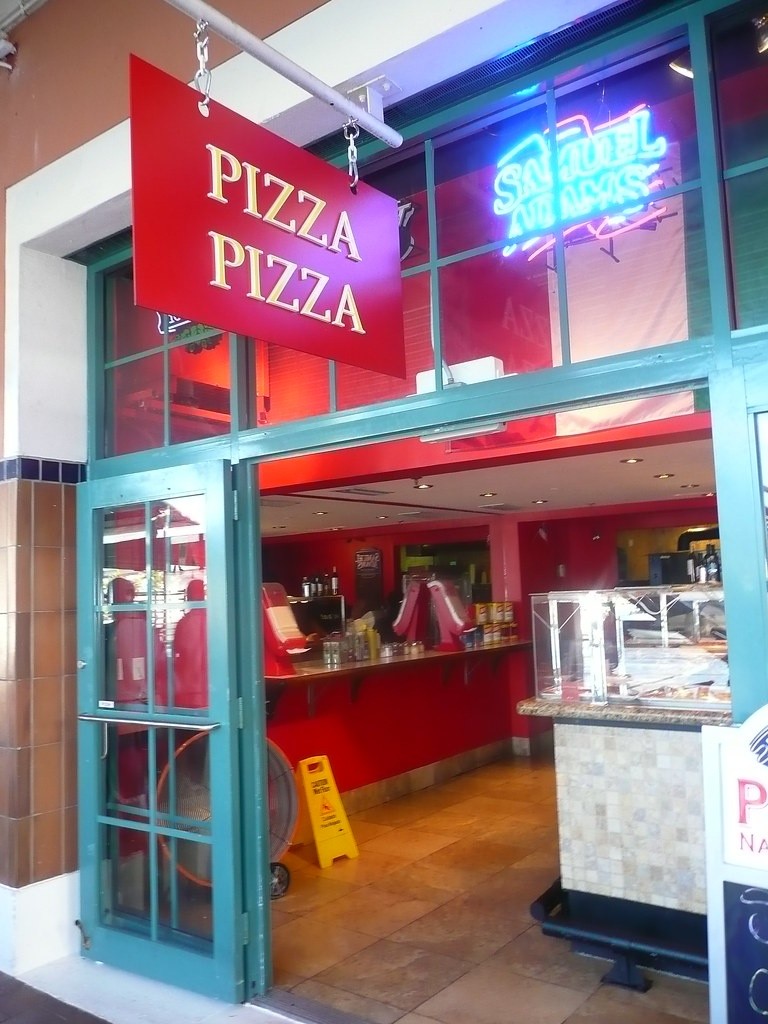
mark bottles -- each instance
[301,566,338,597]
[686,544,720,583]
[381,641,424,657]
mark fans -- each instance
[155,730,300,888]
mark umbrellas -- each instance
[103,501,205,571]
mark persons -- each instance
[174,579,208,785]
[107,578,160,804]
